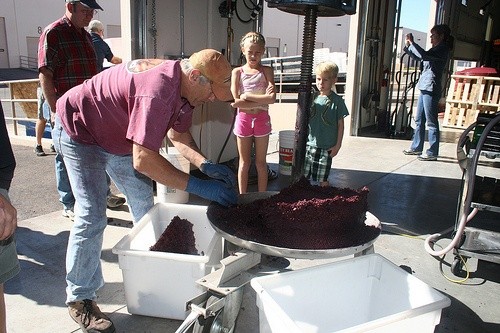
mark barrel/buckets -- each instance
[278,130,295,176]
[156,146,190,203]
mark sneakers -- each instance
[105,193,126,207]
[34,146,45,155]
[50,145,55,152]
[63,207,75,222]
[67,298,115,333]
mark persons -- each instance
[33,79,55,156]
[50,48,233,333]
[84,21,122,72]
[0,100,23,333]
[403,23,454,160]
[301,60,350,187]
[230,32,276,195]
[37,0,126,221]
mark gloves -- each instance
[186,174,239,208]
[199,161,237,187]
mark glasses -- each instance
[80,2,97,14]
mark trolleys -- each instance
[451,105,500,276]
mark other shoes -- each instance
[418,153,436,161]
[403,149,422,154]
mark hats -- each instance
[64,0,103,11]
[189,49,235,104]
